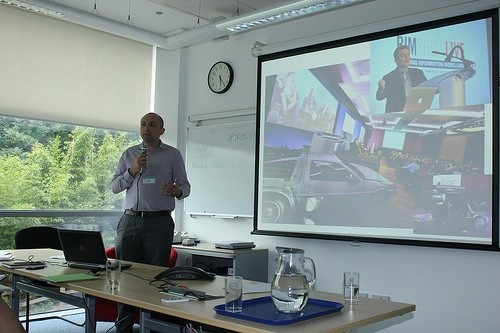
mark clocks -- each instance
[208,61,234,94]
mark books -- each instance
[213,241,255,249]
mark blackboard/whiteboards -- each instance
[185,119,255,219]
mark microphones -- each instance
[431,45,476,66]
[139,143,147,174]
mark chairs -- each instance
[15,226,63,333]
[95,246,178,322]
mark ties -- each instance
[401,70,411,96]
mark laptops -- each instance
[57,229,133,271]
[403,84,437,111]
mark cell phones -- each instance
[27,266,44,270]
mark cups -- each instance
[106,260,120,290]
[224,276,242,312]
[344,272,359,303]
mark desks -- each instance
[0,248,416,333]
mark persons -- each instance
[111,112,190,333]
[0,293,26,333]
[376,46,427,112]
[389,152,480,187]
[276,73,330,127]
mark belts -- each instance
[124,210,171,218]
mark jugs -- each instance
[271,246,316,313]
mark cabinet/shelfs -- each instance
[172,243,269,285]
